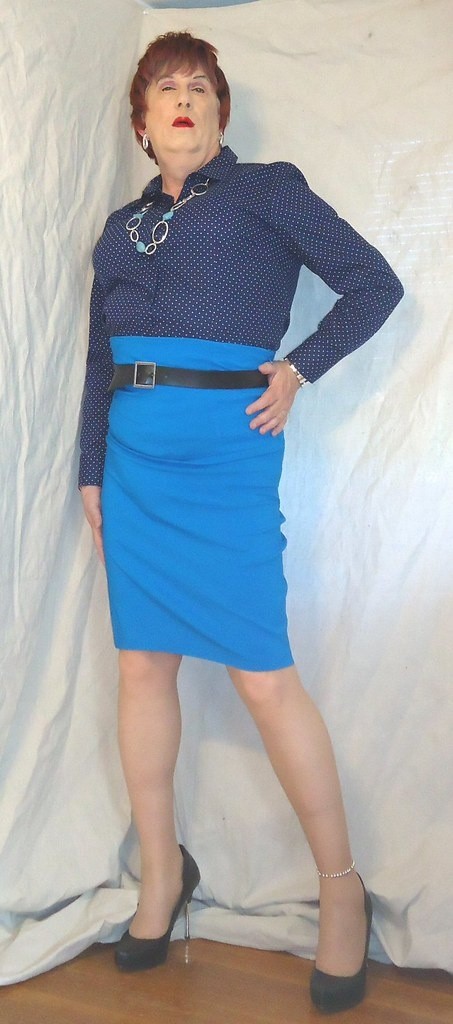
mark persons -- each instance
[76,33,405,1016]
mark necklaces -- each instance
[125,176,212,255]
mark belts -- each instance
[106,360,269,390]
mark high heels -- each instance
[308,872,373,1012]
[114,843,201,971]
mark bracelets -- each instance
[284,357,311,387]
